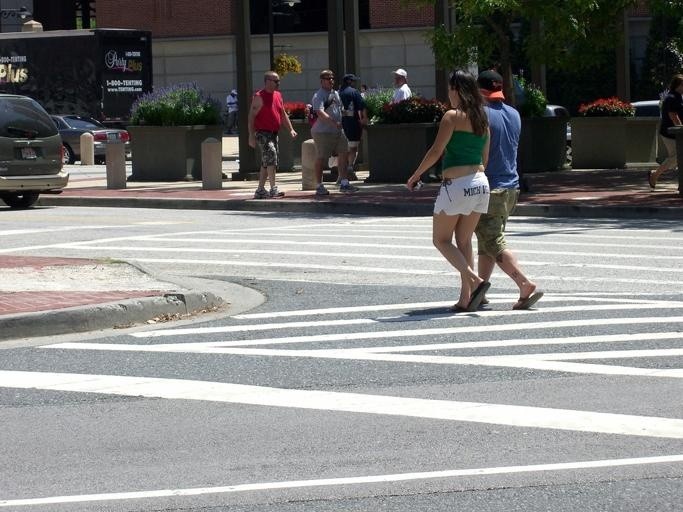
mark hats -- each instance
[474,69,506,102]
[390,68,408,80]
[342,73,361,82]
[232,89,238,96]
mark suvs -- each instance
[0,94,69,209]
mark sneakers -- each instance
[269,188,285,197]
[346,166,359,180]
[339,183,359,193]
[254,189,273,199]
[647,169,656,188]
[315,184,330,195]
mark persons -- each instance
[406,71,490,312]
[474,70,543,309]
[647,74,682,188]
[224,68,413,198]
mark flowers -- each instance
[362,83,417,120]
[284,102,306,119]
[382,97,449,123]
[580,97,636,116]
[127,81,222,126]
[513,68,550,117]
[272,53,300,78]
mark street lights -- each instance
[268,1,301,71]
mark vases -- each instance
[570,117,661,168]
[363,123,432,180]
[276,119,305,171]
[126,125,227,180]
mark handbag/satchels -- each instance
[308,97,335,127]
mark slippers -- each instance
[445,304,477,313]
[465,279,491,312]
[511,291,544,311]
[468,300,492,312]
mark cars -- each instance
[544,105,571,140]
[629,101,662,120]
[46,114,128,163]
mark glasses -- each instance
[267,78,281,84]
[320,77,335,81]
